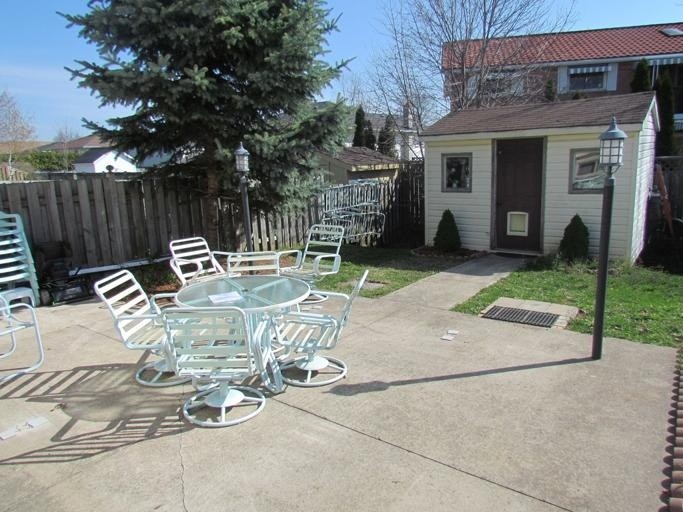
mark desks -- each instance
[173,275,312,394]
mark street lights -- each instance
[591,115,627,359]
[234,140,252,252]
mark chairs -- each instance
[94,268,198,388]
[274,223,346,306]
[268,270,368,388]
[225,251,280,276]
[158,306,266,426]
[167,237,242,287]
[1,211,46,388]
